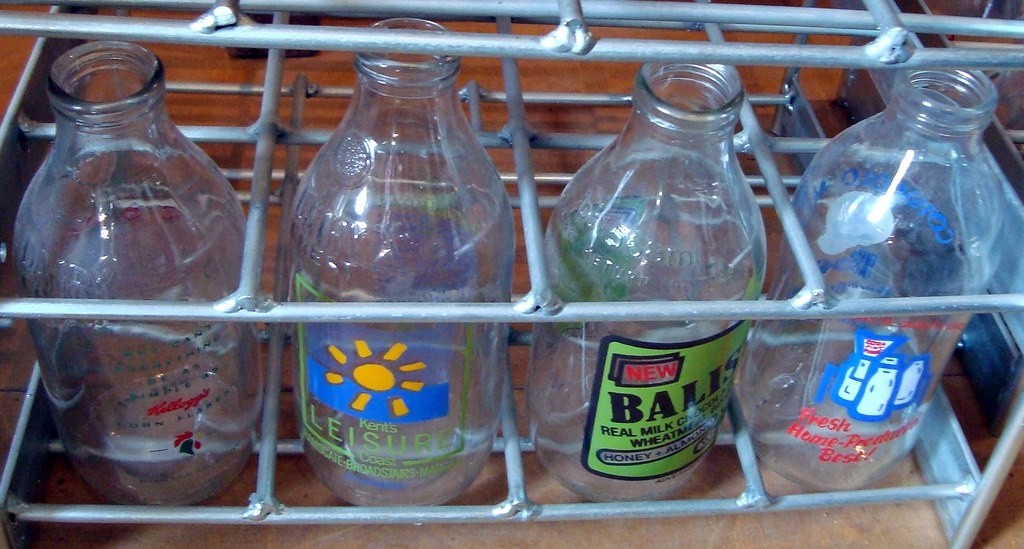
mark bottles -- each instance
[285,19,517,506]
[728,62,1009,489]
[523,63,770,500]
[11,40,263,506]
